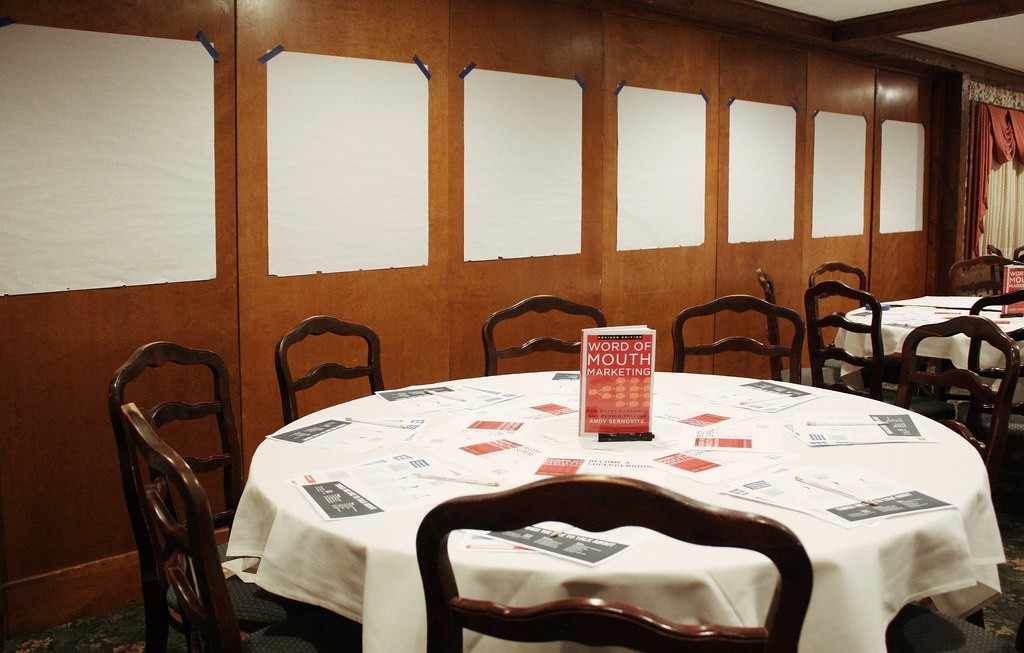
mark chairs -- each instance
[808,261,866,370]
[415,473,814,652]
[482,292,608,376]
[893,314,1021,481]
[805,278,956,420]
[885,601,1024,653]
[757,267,841,387]
[105,339,285,652]
[672,294,806,384]
[949,255,1017,295]
[956,289,1024,438]
[274,314,386,426]
[985,244,1024,300]
[122,401,363,652]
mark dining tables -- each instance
[220,368,1006,652]
[834,295,1024,403]
